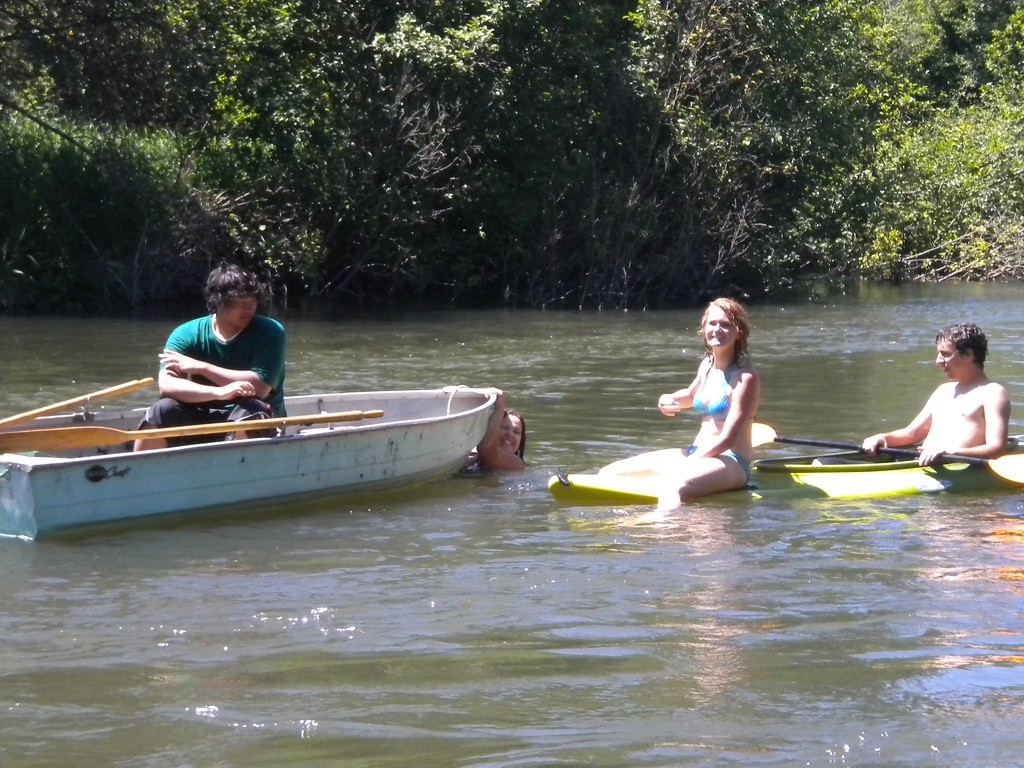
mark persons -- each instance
[130,263,288,453]
[449,386,527,482]
[658,296,761,509]
[862,321,1012,466]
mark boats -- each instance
[545,434,1024,507]
[0,383,499,543]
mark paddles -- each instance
[0,409,385,456]
[749,421,1024,484]
[0,377,155,431]
